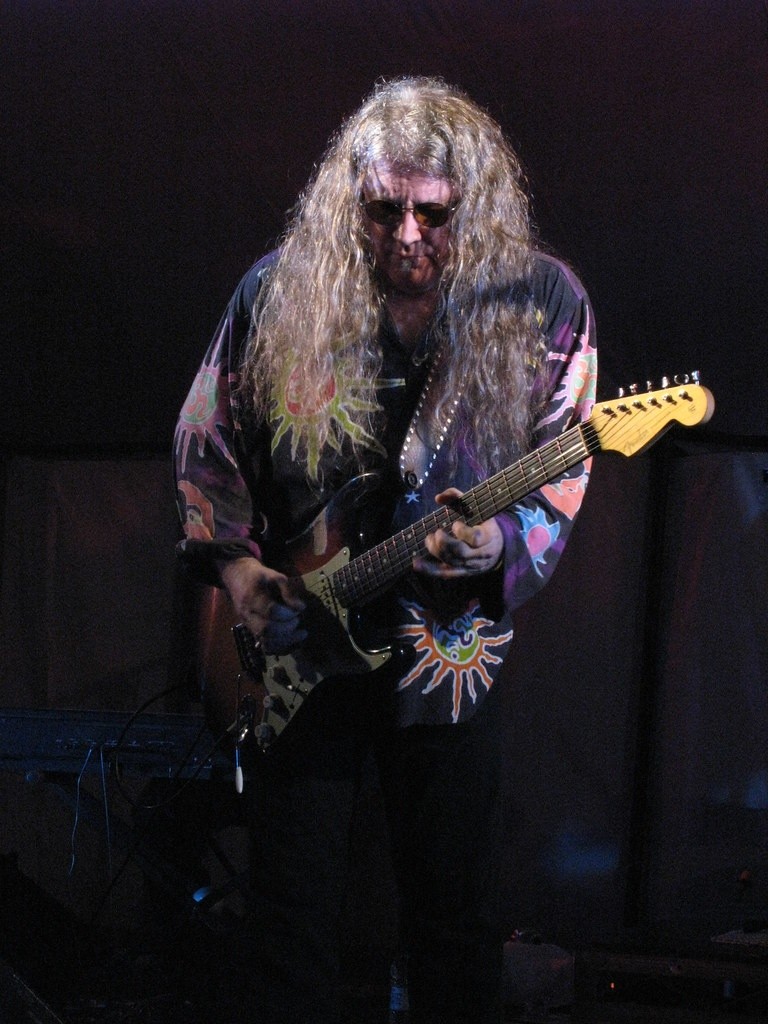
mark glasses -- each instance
[361,199,458,228]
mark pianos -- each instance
[0,706,245,932]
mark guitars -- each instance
[191,368,717,770]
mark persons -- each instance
[170,72,597,1024]
